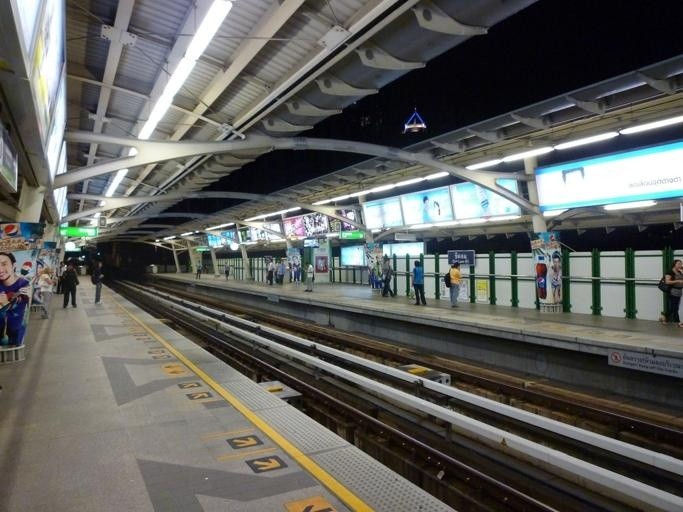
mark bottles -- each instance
[536,256,547,300]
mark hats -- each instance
[451,262,460,268]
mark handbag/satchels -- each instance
[658,278,672,292]
[445,273,452,287]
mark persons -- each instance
[195,260,201,280]
[0,252,46,344]
[40,265,56,318]
[448,261,461,308]
[224,262,230,281]
[263,257,285,285]
[91,260,104,304]
[56,261,79,308]
[305,262,314,292]
[550,252,562,303]
[381,257,396,298]
[422,196,443,221]
[411,260,427,306]
[202,264,208,274]
[288,256,301,282]
[658,260,683,328]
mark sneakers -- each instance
[660,317,683,328]
